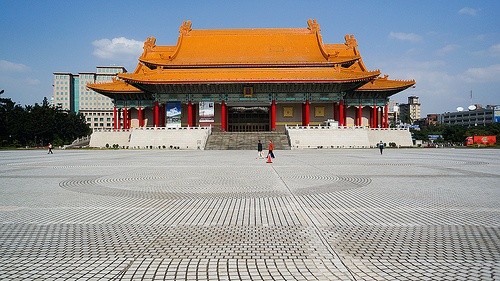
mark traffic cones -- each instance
[266,153,273,163]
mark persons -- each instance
[48,142,53,155]
[266,140,275,158]
[378,140,385,154]
[256,140,265,159]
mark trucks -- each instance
[462,135,496,147]
